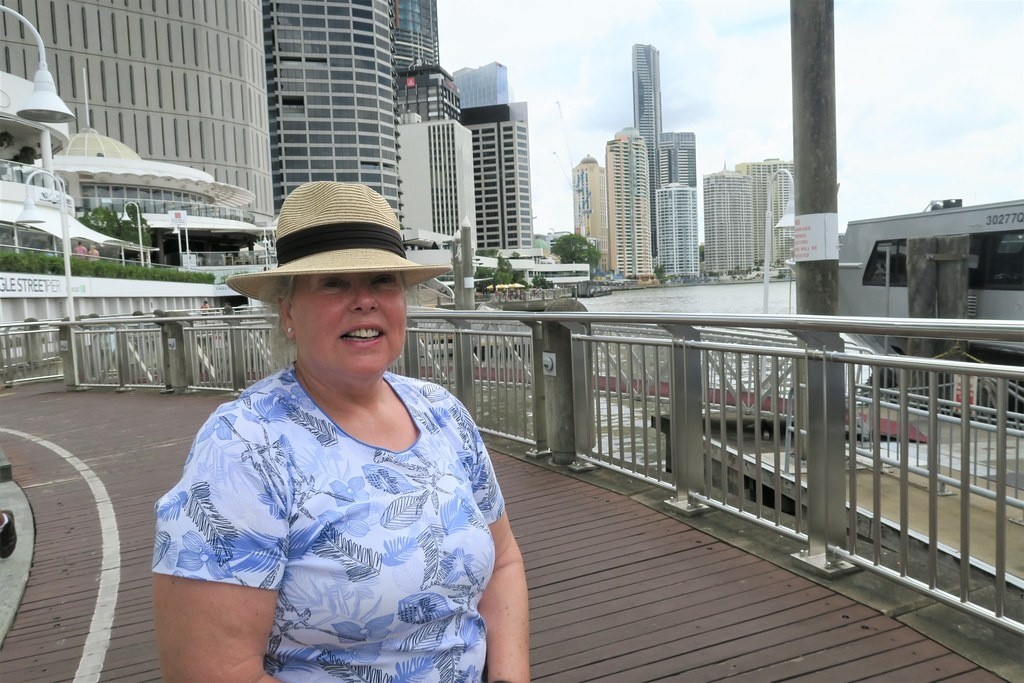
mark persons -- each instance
[150,182,530,683]
[200,301,209,324]
[495,289,527,302]
[72,241,88,260]
[88,245,99,260]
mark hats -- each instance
[225,182,453,305]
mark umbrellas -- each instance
[487,283,525,297]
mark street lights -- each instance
[761,169,802,316]
[17,172,79,322]
[121,202,144,269]
[173,224,192,269]
